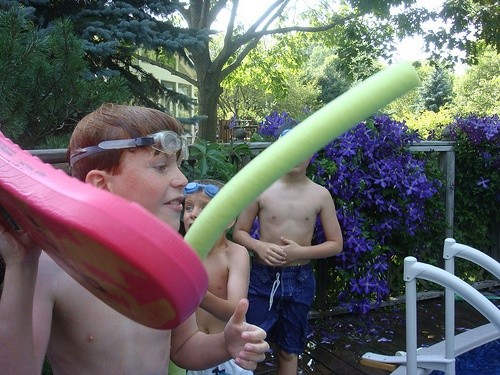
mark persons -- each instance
[185,179,256,375]
[234,129,343,374]
[1,103,270,375]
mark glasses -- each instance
[70,130,189,167]
[183,182,218,198]
